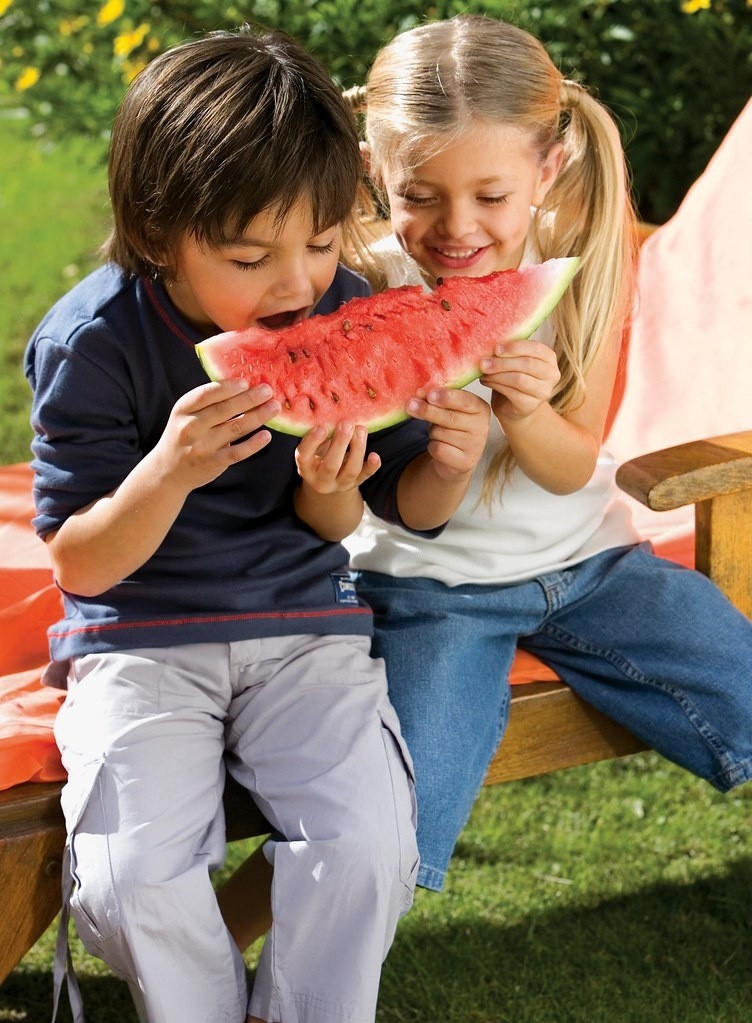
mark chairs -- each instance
[0,218,752,986]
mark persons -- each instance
[216,12,752,956]
[26,19,493,1022]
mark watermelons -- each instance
[198,257,584,436]
